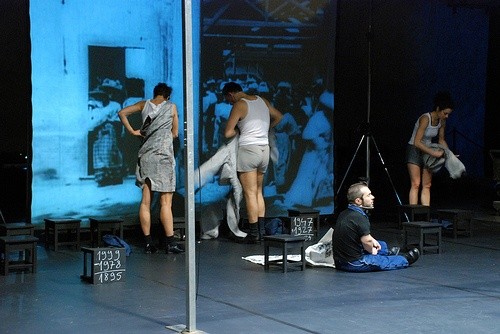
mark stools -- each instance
[262,234,307,273]
[44,218,83,253]
[398,205,432,231]
[0,221,35,256]
[0,235,39,276]
[437,208,473,240]
[402,221,442,255]
[80,247,127,285]
[287,209,320,238]
[172,216,185,239]
[89,217,125,248]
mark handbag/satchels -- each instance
[102,234,130,256]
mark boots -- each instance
[240,222,260,244]
[257,216,266,240]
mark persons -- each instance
[408,98,453,222]
[87,78,335,215]
[332,183,420,272]
[118,83,186,253]
[222,82,283,243]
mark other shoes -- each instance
[167,245,185,254]
[404,248,419,265]
[145,244,159,254]
[388,246,400,257]
